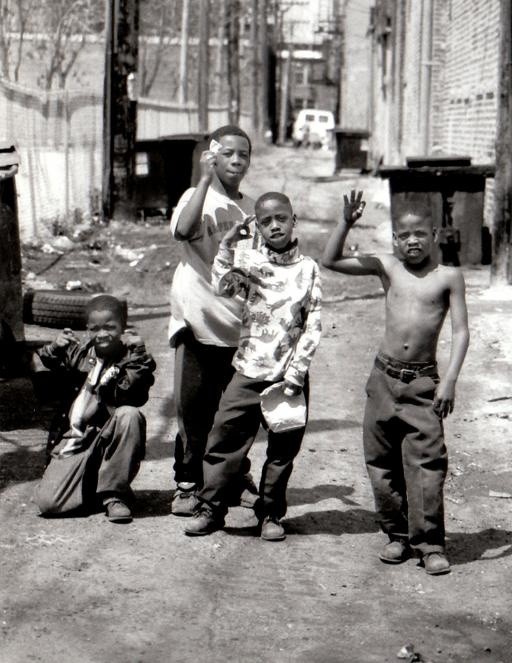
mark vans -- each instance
[292,108,335,150]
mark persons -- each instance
[166,124,269,519]
[181,190,322,543]
[320,188,471,576]
[31,295,155,523]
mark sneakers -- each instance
[103,474,286,541]
[377,542,408,564]
[423,551,451,575]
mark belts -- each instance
[374,358,438,384]
